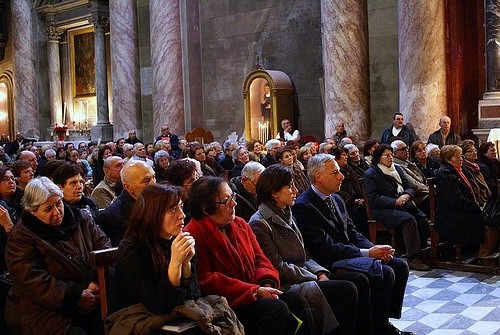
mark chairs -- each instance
[359,178,405,255]
[426,177,499,276]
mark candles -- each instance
[257,121,269,144]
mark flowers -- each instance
[54,125,68,135]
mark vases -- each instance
[58,133,65,141]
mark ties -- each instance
[326,196,337,216]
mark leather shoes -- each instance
[381,323,413,335]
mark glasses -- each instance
[398,146,409,151]
[466,149,477,153]
[382,153,393,158]
[213,191,237,207]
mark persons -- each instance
[154,125,179,143]
[183,176,317,335]
[274,119,302,141]
[380,112,414,148]
[457,139,500,259]
[426,115,461,150]
[124,130,144,145]
[434,144,487,265]
[248,164,371,335]
[0,200,20,335]
[12,131,30,149]
[111,184,237,335]
[0,134,500,249]
[5,176,113,335]
[331,121,349,145]
[292,153,414,335]
[362,144,431,271]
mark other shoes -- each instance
[409,258,429,270]
[462,257,484,266]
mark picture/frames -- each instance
[65,23,111,100]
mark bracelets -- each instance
[253,289,259,299]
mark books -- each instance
[161,319,197,333]
[289,311,303,335]
